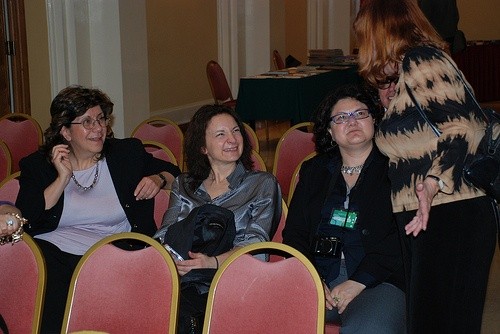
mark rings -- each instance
[50,154,54,156]
[333,297,339,303]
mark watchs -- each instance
[157,173,168,189]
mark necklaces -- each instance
[70,159,99,190]
[341,164,363,175]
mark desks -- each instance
[235,52,367,150]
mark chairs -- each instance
[204,60,271,141]
[272,49,285,71]
[0,112,345,334]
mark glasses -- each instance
[376,76,400,89]
[329,108,371,124]
[69,115,111,130]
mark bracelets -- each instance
[5,211,28,245]
[213,255,219,270]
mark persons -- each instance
[15,86,181,334]
[144,102,282,334]
[281,85,406,334]
[351,0,500,334]
[0,199,31,334]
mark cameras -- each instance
[310,236,343,257]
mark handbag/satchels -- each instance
[463,115,500,206]
[0,212,28,245]
